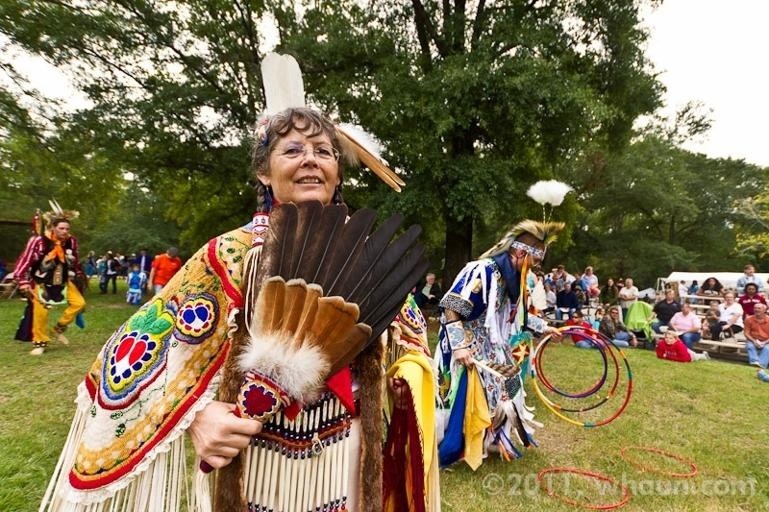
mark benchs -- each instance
[696,338,749,359]
[689,304,712,316]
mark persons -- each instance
[703,291,744,341]
[696,277,725,313]
[127,253,136,271]
[581,266,600,299]
[705,301,735,338]
[677,280,688,305]
[561,311,607,349]
[618,278,639,323]
[669,303,701,350]
[543,283,557,313]
[737,282,769,324]
[100,250,122,294]
[132,249,153,279]
[736,264,766,299]
[744,302,769,369]
[652,289,680,333]
[550,275,558,293]
[14,209,86,356]
[554,282,578,328]
[643,294,650,303]
[146,247,183,296]
[599,307,640,348]
[571,272,589,307]
[96,255,103,280]
[656,330,711,362]
[114,252,126,280]
[688,280,699,304]
[125,264,146,305]
[527,265,548,313]
[592,308,605,331]
[411,272,442,308]
[38,105,443,512]
[551,265,566,289]
[434,219,565,473]
[600,278,618,307]
[545,274,551,282]
[87,250,97,279]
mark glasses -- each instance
[277,142,342,165]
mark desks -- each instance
[686,295,739,306]
